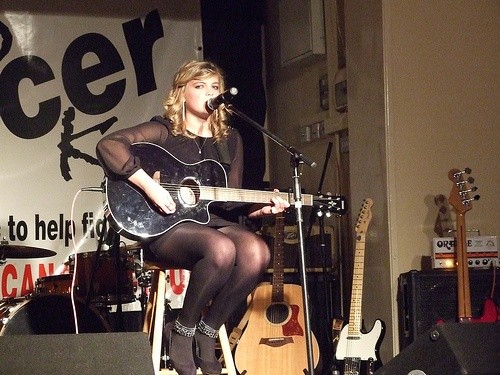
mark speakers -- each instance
[0,332,154,375]
[373,269,500,375]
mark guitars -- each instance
[102,142,349,242]
[329,198,386,375]
[234,212,323,375]
[436,168,500,325]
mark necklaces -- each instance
[186,126,210,154]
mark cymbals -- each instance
[0,244,57,259]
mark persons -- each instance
[96,60,270,375]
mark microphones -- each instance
[207,87,238,110]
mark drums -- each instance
[36,274,73,296]
[69,249,136,306]
[0,296,111,335]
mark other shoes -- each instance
[194,321,222,375]
[164,317,197,375]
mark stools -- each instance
[142,261,236,375]
